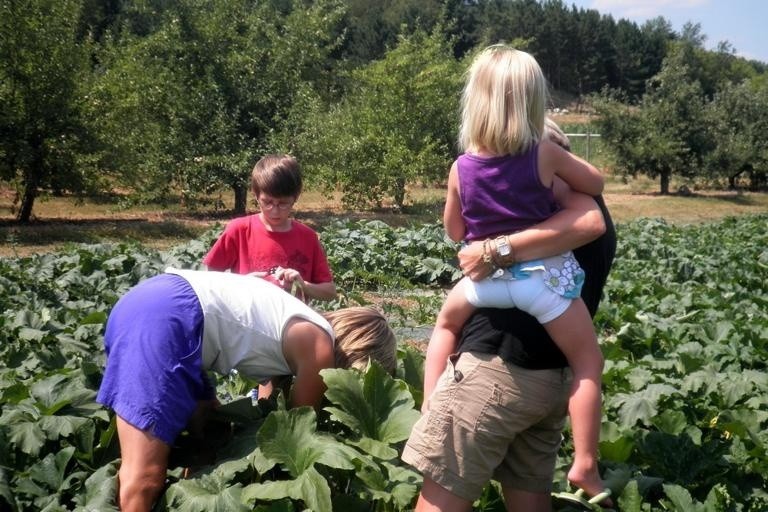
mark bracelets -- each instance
[481,235,500,271]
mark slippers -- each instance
[558,487,612,512]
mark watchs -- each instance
[496,235,513,269]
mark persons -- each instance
[420,42,614,508]
[95,269,398,512]
[400,114,618,512]
[206,153,338,410]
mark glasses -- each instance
[261,199,294,210]
